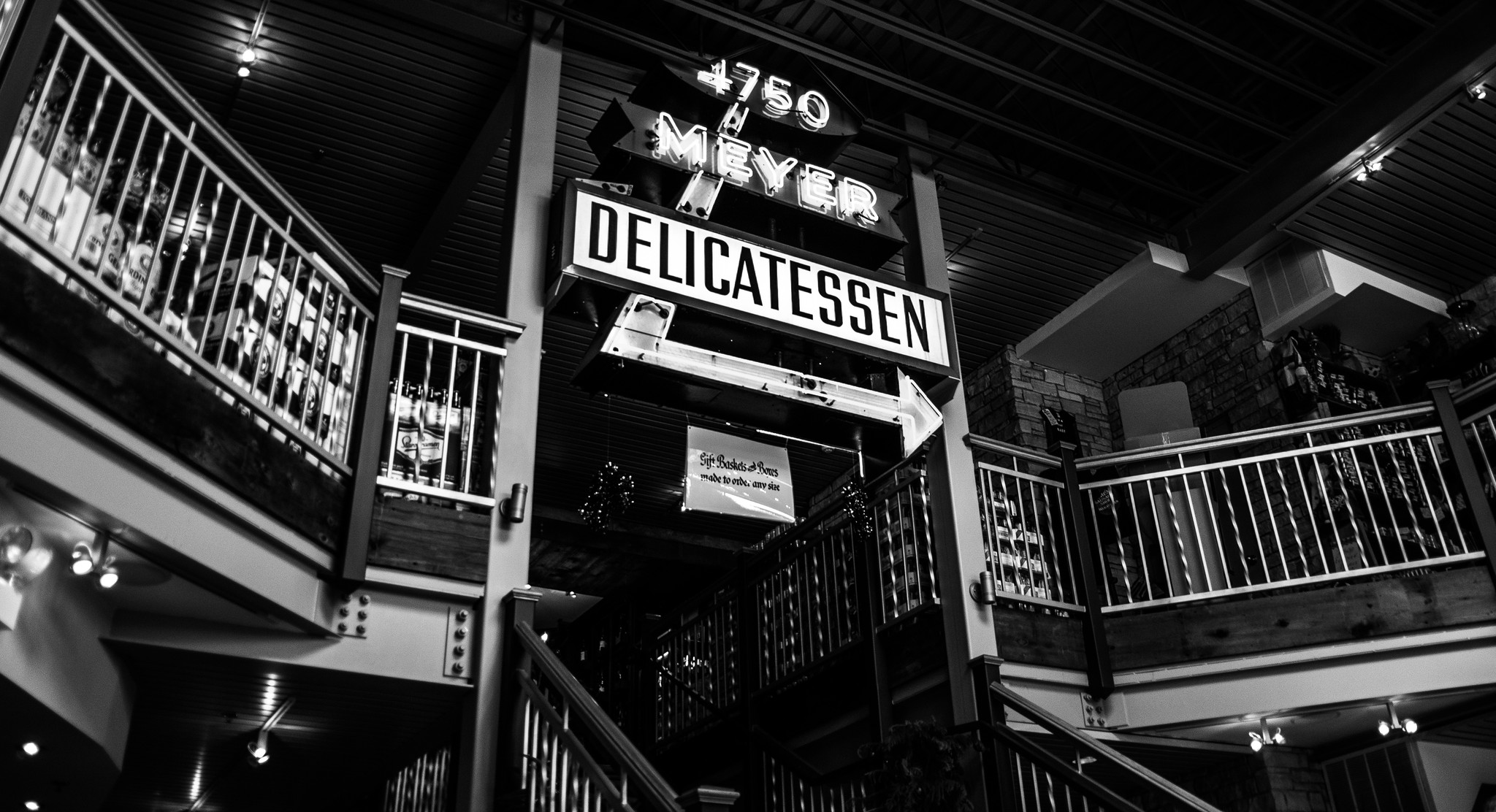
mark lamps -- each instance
[244,731,270,767]
[1246,720,1288,753]
[1354,157,1383,182]
[1375,700,1419,737]
[1,522,54,589]
[233,41,257,80]
[65,539,123,591]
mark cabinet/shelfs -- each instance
[1268,339,1464,574]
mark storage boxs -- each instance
[873,465,1055,619]
[0,100,479,512]
